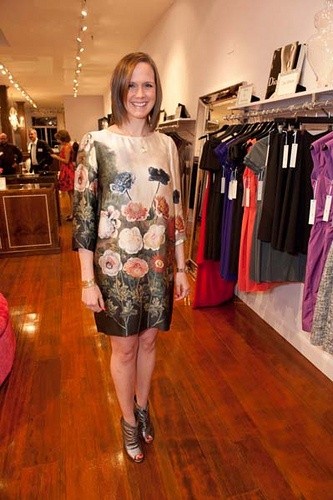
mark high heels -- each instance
[133,395,155,444]
[120,417,145,463]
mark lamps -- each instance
[81,0,88,31]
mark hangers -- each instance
[199,100,333,149]
[161,125,192,146]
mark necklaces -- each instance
[123,126,150,153]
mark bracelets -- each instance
[81,278,97,289]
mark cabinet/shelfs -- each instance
[0,172,62,258]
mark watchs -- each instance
[177,265,188,274]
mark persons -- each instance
[0,133,23,175]
[50,130,75,221]
[74,51,191,462]
[28,130,54,174]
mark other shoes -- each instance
[66,217,73,221]
[65,215,71,218]
